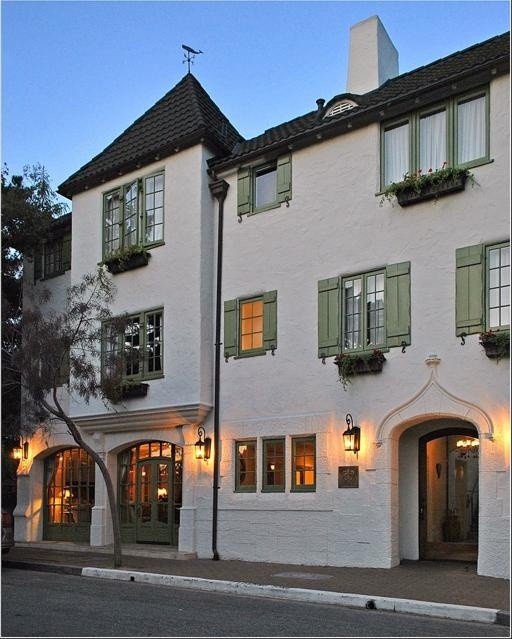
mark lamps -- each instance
[194,426,211,461]
[12,429,29,462]
[342,413,360,454]
